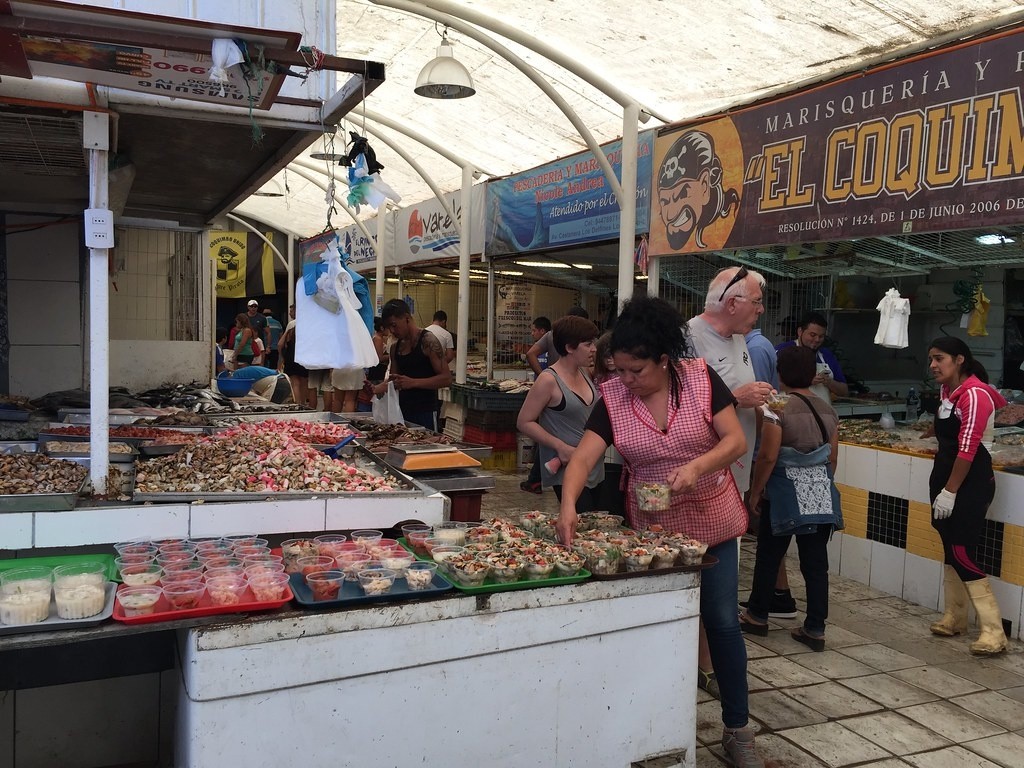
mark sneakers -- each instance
[723,728,765,768]
[738,597,798,618]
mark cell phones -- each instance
[818,370,832,376]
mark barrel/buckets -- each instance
[516,432,535,467]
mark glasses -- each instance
[719,265,748,301]
[250,305,257,307]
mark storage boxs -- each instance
[438,380,527,471]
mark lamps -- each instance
[414,31,476,100]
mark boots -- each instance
[963,577,1008,655]
[930,563,968,636]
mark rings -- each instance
[763,395,765,399]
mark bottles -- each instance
[905,388,918,422]
[879,401,896,429]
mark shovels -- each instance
[320,434,356,460]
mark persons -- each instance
[216,300,390,413]
[774,314,848,406]
[362,299,453,434]
[532,317,551,383]
[590,331,620,397]
[555,298,766,768]
[520,306,590,494]
[739,345,845,652]
[926,336,1009,655]
[517,316,605,514]
[678,265,774,563]
[745,328,780,536]
[424,310,454,364]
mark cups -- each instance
[280,538,321,572]
[314,533,347,562]
[379,551,439,591]
[331,543,366,559]
[401,510,709,587]
[528,372,535,382]
[113,531,290,617]
[351,530,399,565]
[352,560,397,597]
[306,571,345,602]
[296,555,335,584]
[335,553,372,581]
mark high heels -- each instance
[791,627,825,652]
[738,611,769,636]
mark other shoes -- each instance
[520,478,543,494]
[699,669,721,700]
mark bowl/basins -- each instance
[635,481,672,511]
[215,376,255,397]
[767,395,790,410]
[51,561,108,620]
[0,566,52,626]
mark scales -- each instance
[383,443,482,474]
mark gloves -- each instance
[932,488,957,519]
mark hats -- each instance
[248,300,258,306]
